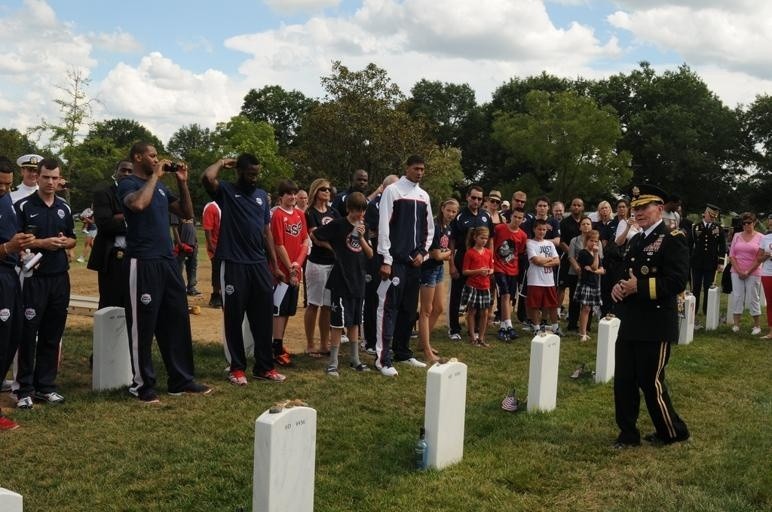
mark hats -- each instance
[488,190,502,201]
[16,153,45,168]
[628,183,669,208]
[501,200,510,207]
[705,203,721,218]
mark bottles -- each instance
[415,426,428,470]
[21,248,34,266]
[162,162,180,173]
[353,216,365,237]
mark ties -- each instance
[706,223,709,229]
[637,232,646,246]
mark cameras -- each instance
[162,162,181,172]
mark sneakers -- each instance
[731,325,772,340]
[272,347,293,367]
[340,334,399,376]
[447,330,489,348]
[400,357,427,368]
[0,416,20,430]
[253,368,287,381]
[35,391,64,403]
[307,350,343,376]
[168,382,213,396]
[17,396,34,409]
[496,320,587,342]
[226,369,248,386]
[137,386,160,403]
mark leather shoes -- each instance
[645,427,690,445]
[608,437,641,452]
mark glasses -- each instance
[471,196,482,201]
[514,198,525,203]
[491,200,500,204]
[319,186,332,192]
[742,221,752,225]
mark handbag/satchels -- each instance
[721,262,733,295]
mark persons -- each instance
[450,186,643,348]
[201,152,463,385]
[80,142,213,404]
[0,153,77,432]
[603,185,690,444]
[664,193,772,339]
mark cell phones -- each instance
[631,213,634,219]
[24,223,33,234]
